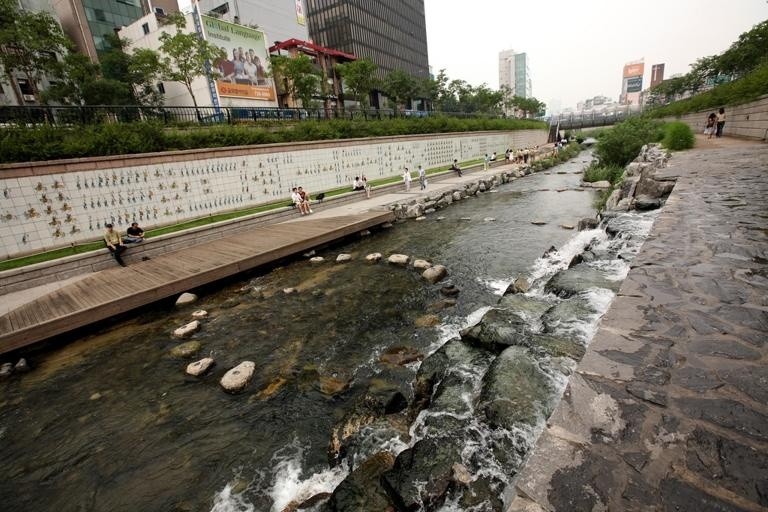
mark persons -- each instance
[353,177,364,190]
[298,187,313,214]
[418,166,426,190]
[125,223,145,243]
[717,107,726,137]
[103,224,127,268]
[404,168,411,191]
[488,152,497,161]
[292,187,305,215]
[704,113,716,139]
[452,159,462,177]
[215,46,265,85]
[483,154,490,171]
[504,138,566,170]
[361,177,370,198]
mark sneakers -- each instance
[119,263,127,267]
[110,251,115,257]
[299,208,314,216]
[140,257,151,261]
